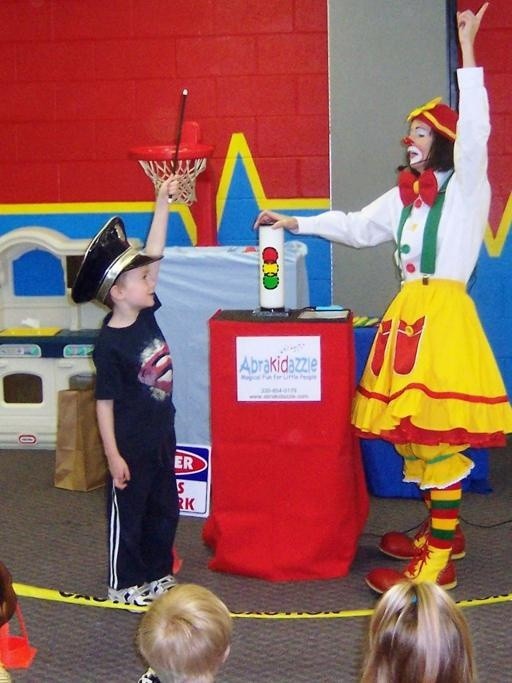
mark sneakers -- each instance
[108,572,178,613]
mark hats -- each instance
[71,216,164,305]
[413,103,459,144]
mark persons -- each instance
[359,582,476,681]
[137,583,232,682]
[252,0,512,594]
[70,174,181,606]
[2,560,18,683]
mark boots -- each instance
[365,541,458,596]
[378,520,466,561]
